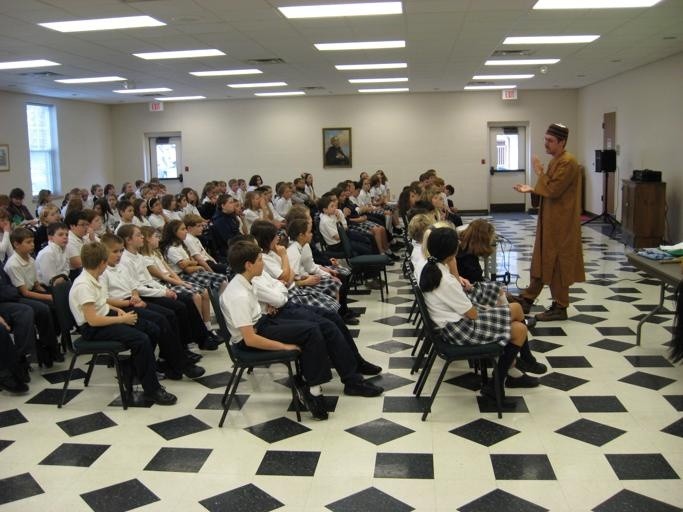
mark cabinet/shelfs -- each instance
[620,178,682,255]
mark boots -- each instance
[514,318,548,376]
[478,341,519,409]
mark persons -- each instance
[324,134,350,164]
[398,169,539,402]
[218,168,404,419]
[1,172,320,405]
[508,122,586,321]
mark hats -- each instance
[544,121,570,140]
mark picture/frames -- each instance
[322,127,353,170]
[1,144,10,170]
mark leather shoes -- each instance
[506,293,534,315]
[141,384,178,406]
[534,301,569,321]
[116,359,137,394]
[154,326,226,381]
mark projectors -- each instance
[456,224,470,239]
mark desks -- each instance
[624,248,682,353]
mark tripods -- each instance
[581,172,621,234]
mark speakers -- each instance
[595,150,616,172]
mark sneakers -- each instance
[340,308,361,325]
[35,340,63,369]
[341,356,386,398]
[300,380,329,421]
[504,355,540,389]
[364,276,387,290]
[385,226,406,266]
